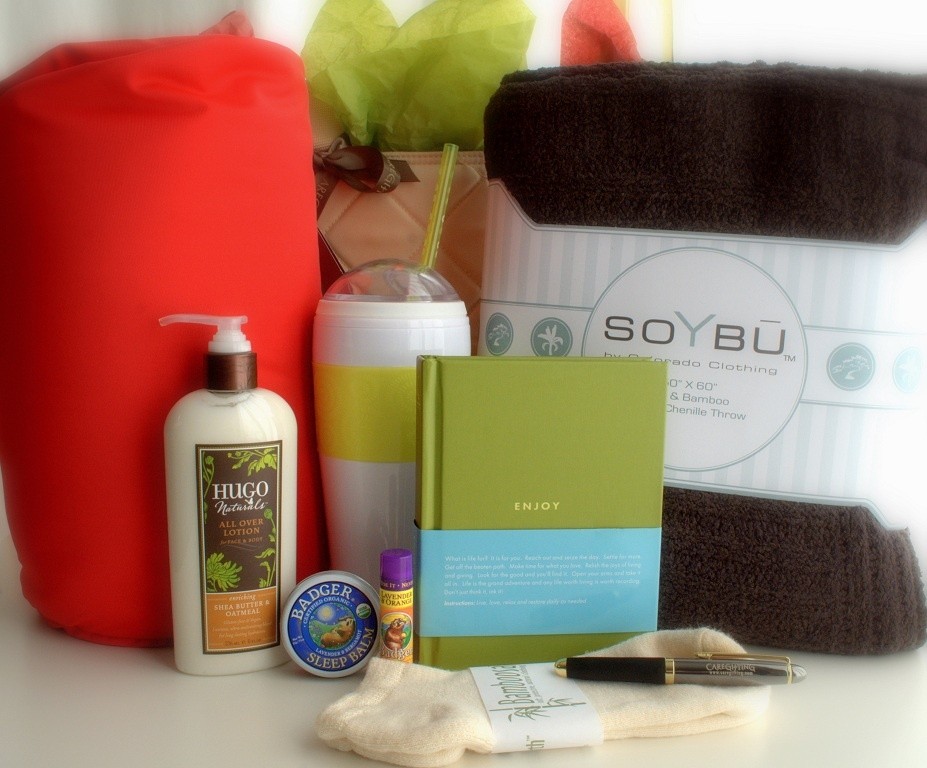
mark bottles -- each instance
[378,548,413,666]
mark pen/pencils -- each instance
[555,652,808,687]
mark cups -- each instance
[310,261,470,593]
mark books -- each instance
[412,355,666,670]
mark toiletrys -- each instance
[157,313,297,677]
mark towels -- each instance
[479,62,927,656]
[1,32,324,652]
